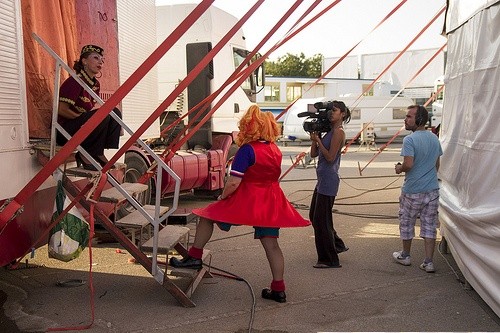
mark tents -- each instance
[436,1,500,316]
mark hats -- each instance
[81,44,104,57]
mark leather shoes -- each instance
[169,254,202,272]
[262,288,287,303]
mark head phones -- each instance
[416,106,425,126]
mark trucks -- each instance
[0,0,266,268]
[282,71,443,146]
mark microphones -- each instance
[298,112,311,117]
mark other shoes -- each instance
[337,247,349,254]
[313,263,342,268]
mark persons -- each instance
[309,101,350,268]
[169,106,312,303]
[393,105,443,272]
[49,45,122,171]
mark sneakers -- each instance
[393,251,411,266]
[420,260,434,272]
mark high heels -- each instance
[75,152,98,171]
[89,152,116,169]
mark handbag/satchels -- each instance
[48,180,92,262]
